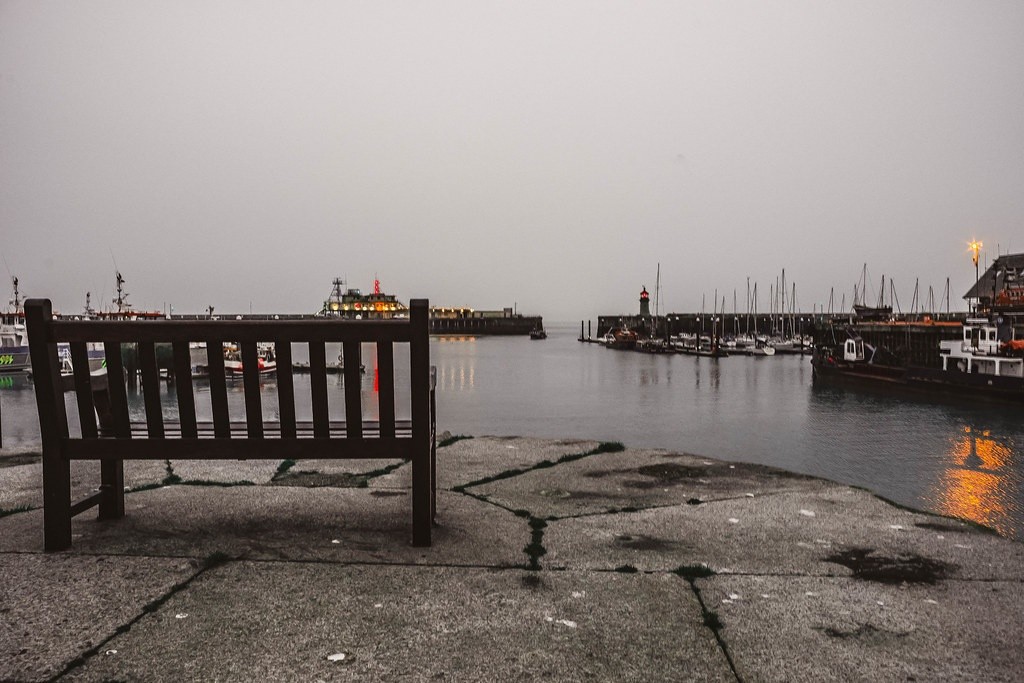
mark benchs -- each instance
[23,298,437,553]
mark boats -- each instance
[530,321,548,339]
[809,341,990,413]
[1,273,279,391]
[293,343,366,374]
[314,276,407,320]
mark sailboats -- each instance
[602,245,1000,358]
